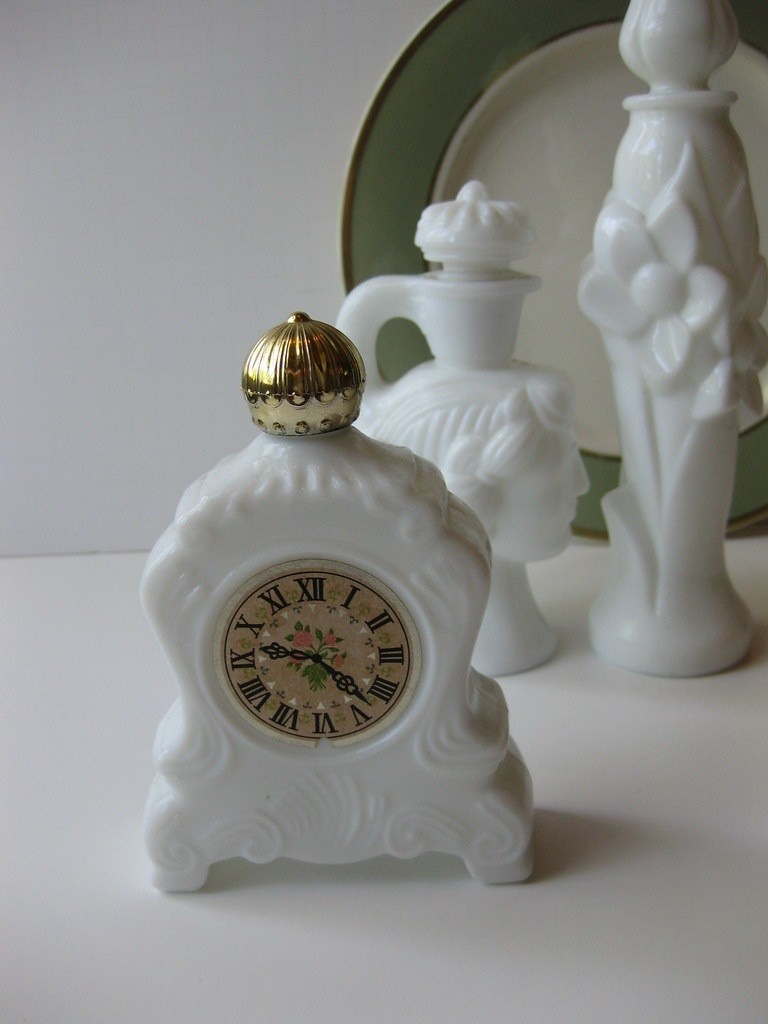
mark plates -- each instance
[337,1,767,542]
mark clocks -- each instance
[139,311,534,895]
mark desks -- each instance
[0,537,768,1024]
